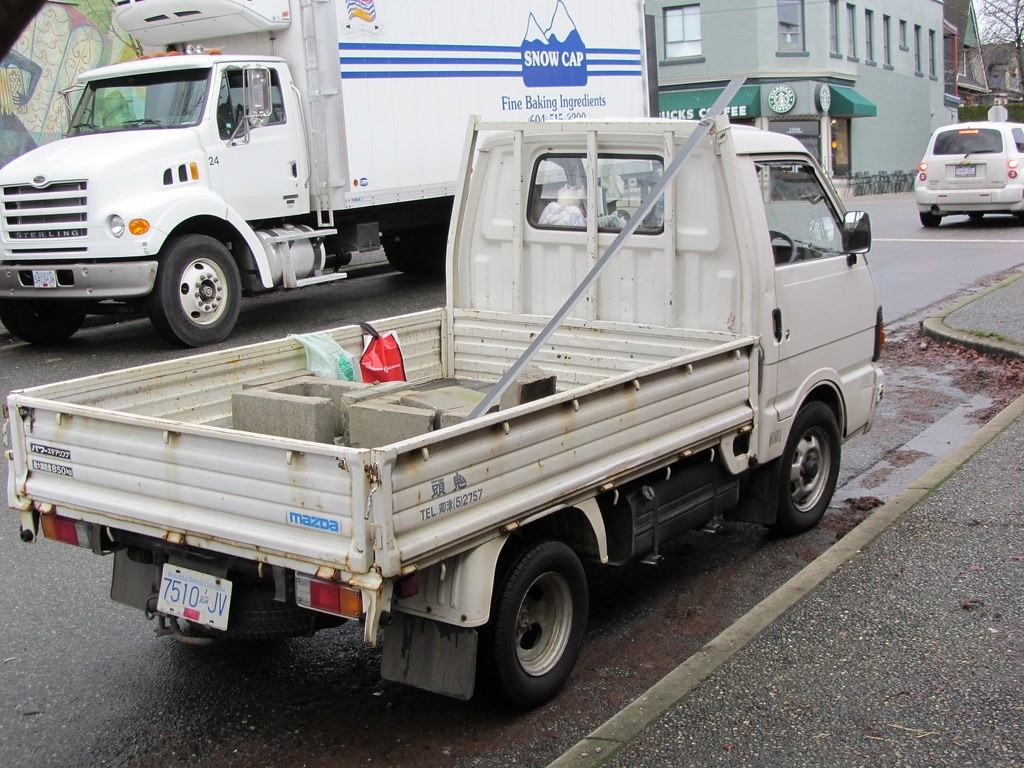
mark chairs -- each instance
[844,170,916,198]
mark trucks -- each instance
[0,0,655,349]
[0,117,883,721]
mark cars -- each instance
[913,121,1024,227]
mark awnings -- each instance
[658,86,762,120]
[828,86,878,118]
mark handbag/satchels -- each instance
[356,321,406,384]
[287,333,363,384]
